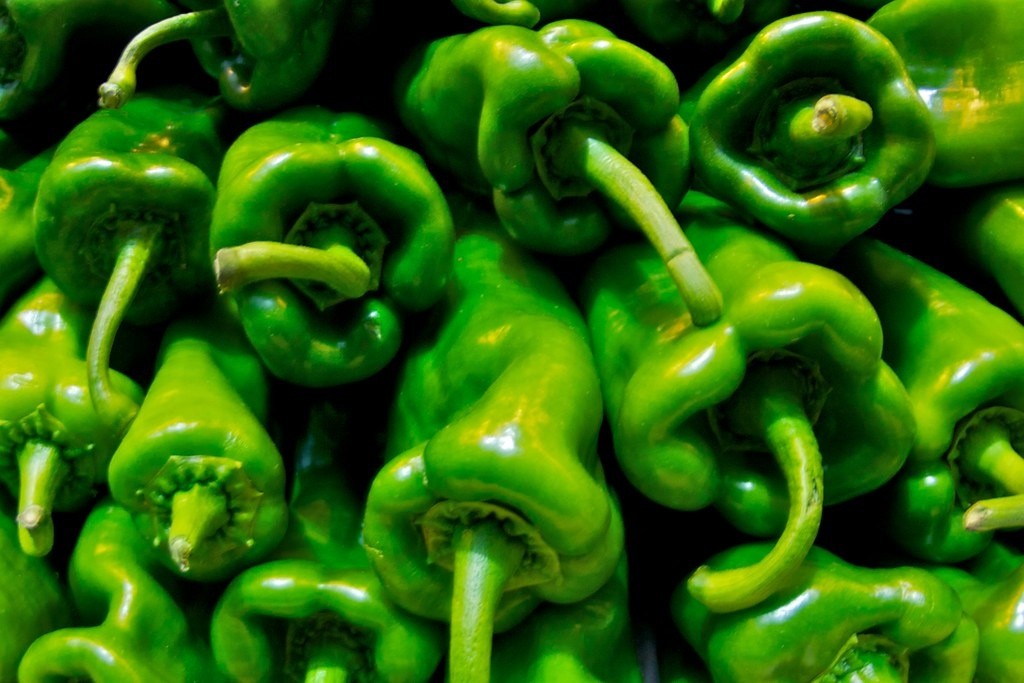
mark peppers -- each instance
[0,0,1024,683]
